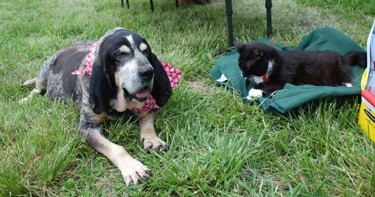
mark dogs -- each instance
[17,27,173,188]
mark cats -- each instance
[232,39,366,106]
